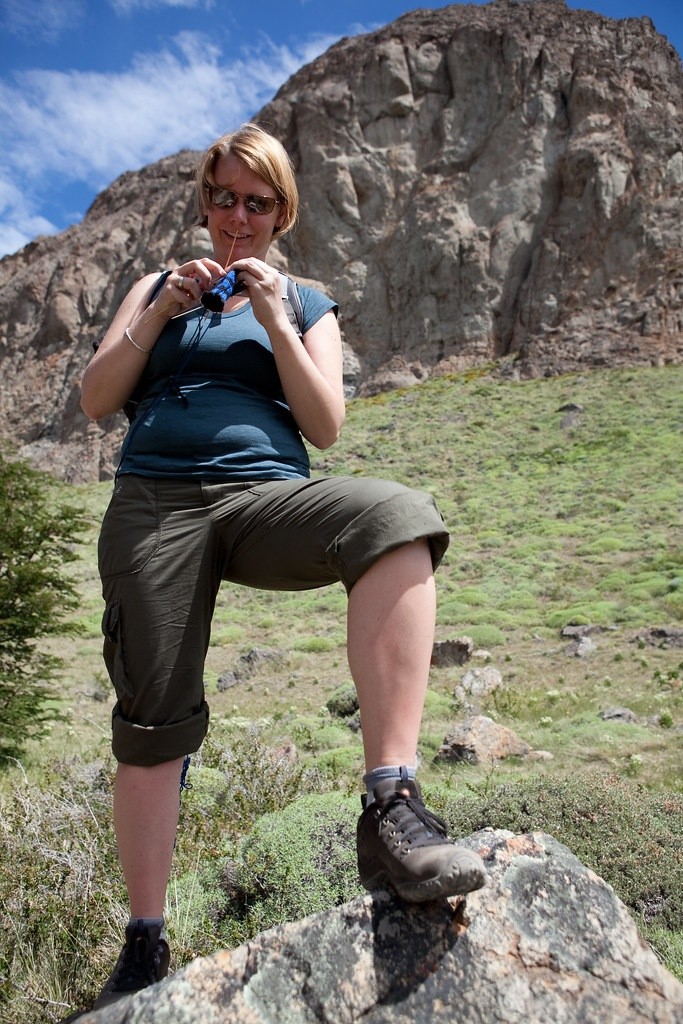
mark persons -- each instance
[80,123,486,1012]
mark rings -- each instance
[178,276,183,288]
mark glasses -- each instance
[209,185,281,215]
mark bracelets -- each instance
[124,328,152,355]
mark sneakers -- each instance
[357,765,487,903]
[93,919,170,1009]
